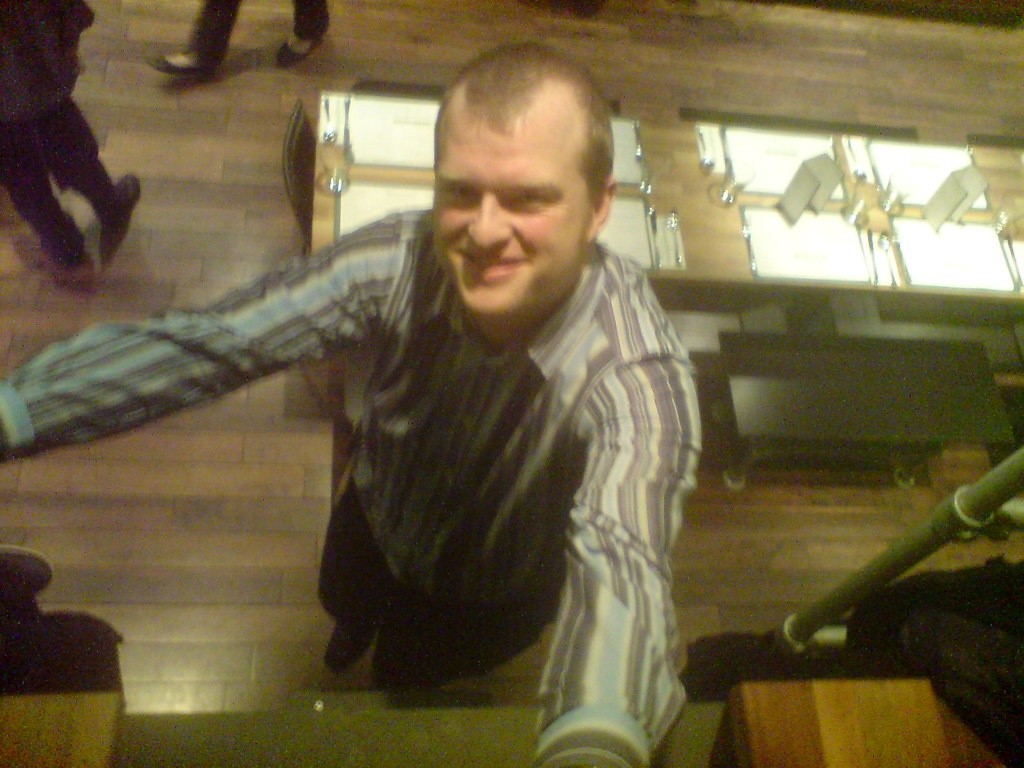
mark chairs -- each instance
[281,98,315,255]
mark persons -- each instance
[0,0,332,264]
[0,40,703,768]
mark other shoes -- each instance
[103,173,139,226]
[276,33,320,62]
[38,237,82,265]
[146,50,215,76]
[325,617,378,671]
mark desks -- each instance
[311,92,1024,377]
[719,332,1017,495]
